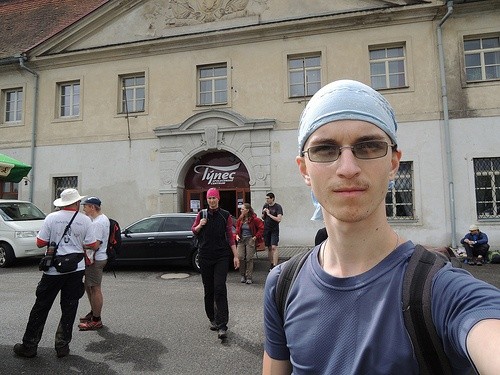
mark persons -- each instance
[78,196,111,331]
[12,187,98,359]
[261,192,283,275]
[235,202,265,283]
[315,227,330,246]
[192,187,240,341]
[263,78,500,375]
[460,223,490,266]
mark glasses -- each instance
[303,140,395,163]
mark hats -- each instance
[207,188,219,198]
[53,188,87,207]
[468,224,479,231]
[81,197,101,206]
[299,79,397,156]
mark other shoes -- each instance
[469,258,483,265]
[269,264,275,271]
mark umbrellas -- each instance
[0,154,32,184]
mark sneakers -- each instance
[14,343,37,358]
[241,277,253,284]
[211,322,216,330]
[57,347,70,357]
[217,328,227,339]
[78,312,103,331]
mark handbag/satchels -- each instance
[55,254,79,273]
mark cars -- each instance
[0,199,50,268]
[102,213,240,274]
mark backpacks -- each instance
[106,219,122,259]
